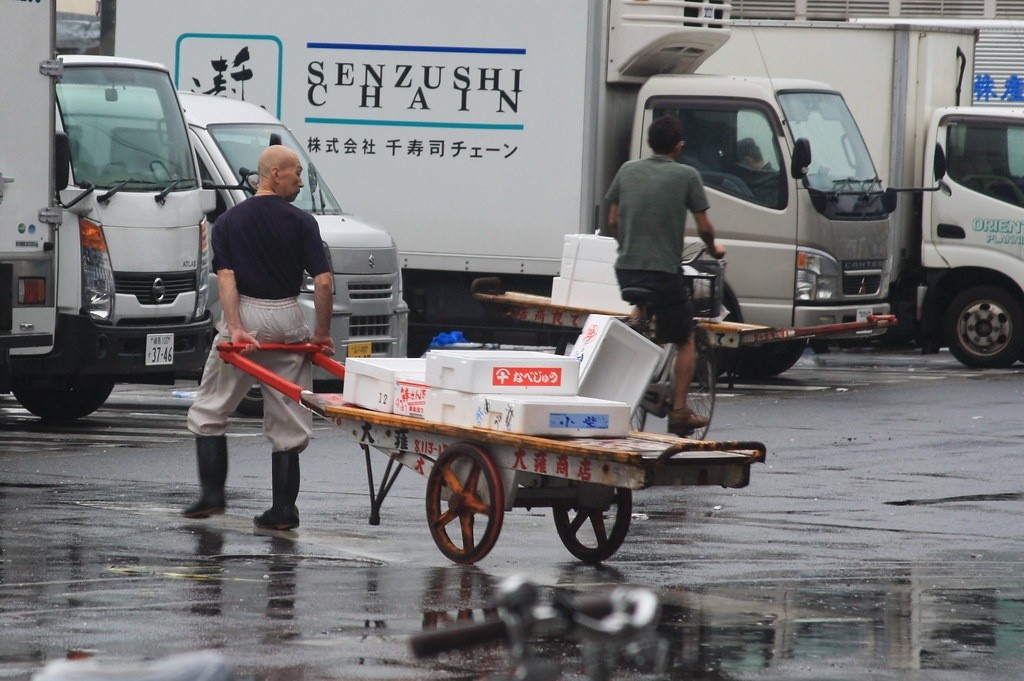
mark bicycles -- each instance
[619,247,727,441]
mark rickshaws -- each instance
[216,341,766,565]
[470,277,899,381]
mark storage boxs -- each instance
[340,314,666,439]
[551,233,636,313]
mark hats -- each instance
[738,138,759,155]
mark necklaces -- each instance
[257,189,274,194]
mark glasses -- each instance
[680,140,685,146]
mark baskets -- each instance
[682,259,727,317]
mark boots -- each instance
[184,436,227,519]
[254,451,300,530]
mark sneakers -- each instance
[668,406,709,432]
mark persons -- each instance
[605,115,725,433]
[735,137,775,170]
[180,145,336,530]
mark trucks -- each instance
[693,19,1024,369]
[1,0,283,421]
[102,1,947,381]
[56,84,409,422]
